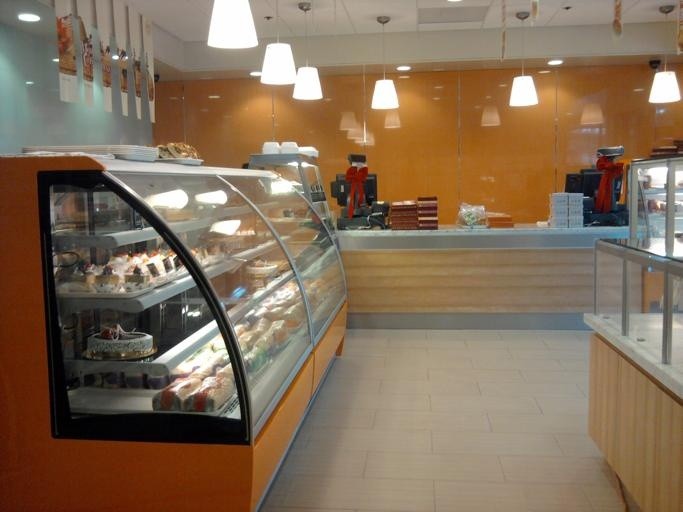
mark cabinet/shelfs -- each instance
[247,155,339,254]
[1,158,349,512]
[581,156,683,512]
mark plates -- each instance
[22,145,159,162]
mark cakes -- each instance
[66,244,209,293]
[246,261,278,278]
[85,324,157,358]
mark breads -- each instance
[159,141,201,160]
[459,211,486,225]
[151,278,330,412]
[638,175,683,213]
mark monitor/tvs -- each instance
[580,165,624,210]
[336,173,378,207]
[564,172,581,191]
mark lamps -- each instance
[260,1,296,86]
[207,0,258,50]
[647,5,681,103]
[579,103,605,126]
[507,10,539,107]
[339,111,376,147]
[291,2,323,101]
[371,15,399,110]
[382,112,402,129]
[480,105,500,127]
